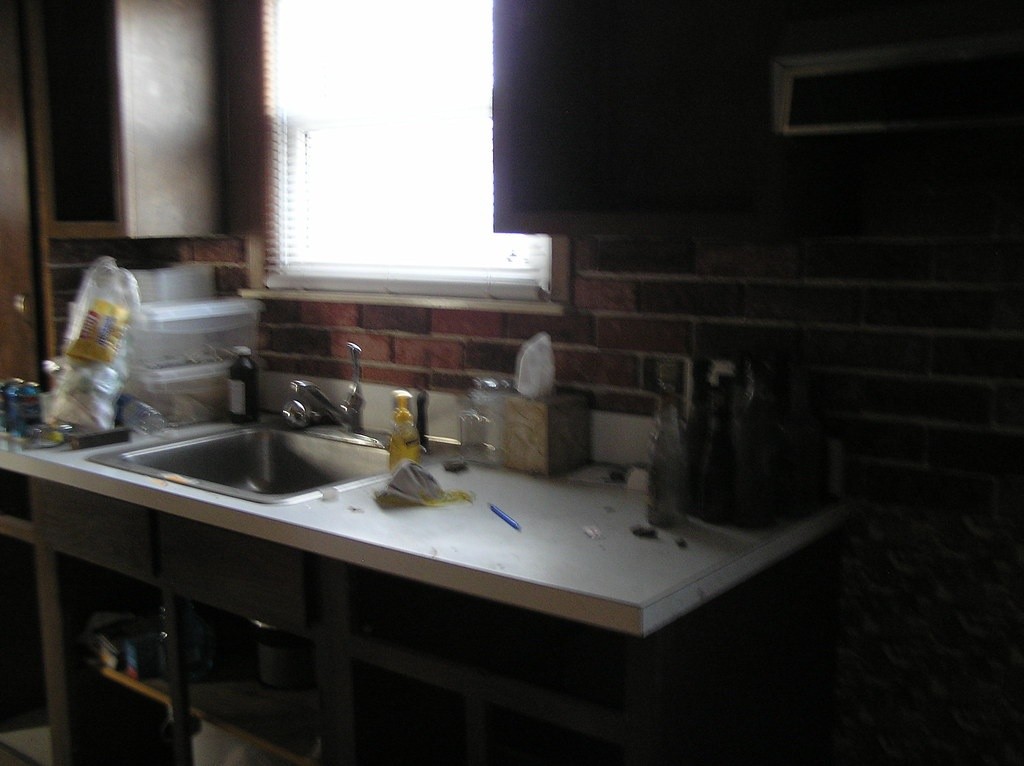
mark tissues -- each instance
[504,331,592,480]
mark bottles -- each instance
[389,409,420,473]
[115,394,164,438]
[645,366,689,526]
[465,377,514,467]
[227,345,258,425]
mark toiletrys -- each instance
[227,346,258,426]
[389,389,420,471]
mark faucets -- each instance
[289,341,367,435]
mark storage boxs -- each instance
[128,296,262,429]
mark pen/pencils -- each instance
[488,502,522,531]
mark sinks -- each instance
[83,424,436,507]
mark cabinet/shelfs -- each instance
[30,0,228,241]
[0,468,839,766]
[493,0,1024,233]
